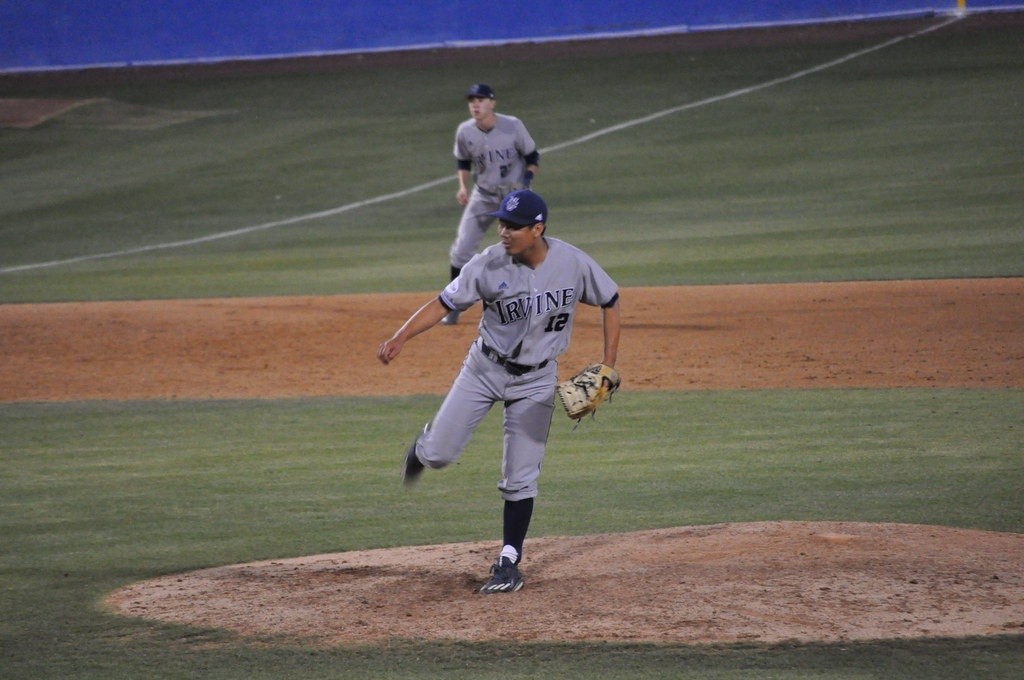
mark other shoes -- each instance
[441,310,459,324]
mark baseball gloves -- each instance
[494,182,528,202]
[556,362,622,432]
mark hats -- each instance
[485,190,547,226]
[465,84,496,99]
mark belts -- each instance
[475,184,496,196]
[482,343,548,376]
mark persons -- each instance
[378,190,621,596]
[441,83,540,325]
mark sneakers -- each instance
[479,556,525,595]
[403,422,433,482]
